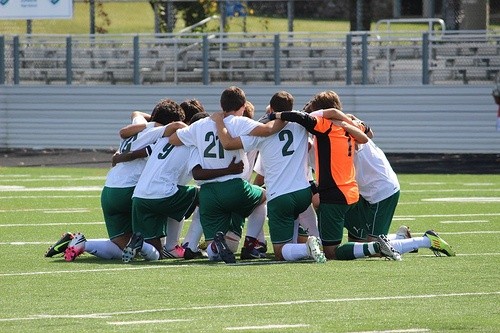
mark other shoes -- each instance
[181,242,189,248]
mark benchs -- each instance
[3,37,500,84]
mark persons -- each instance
[44,85,455,261]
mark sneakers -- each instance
[122,231,143,263]
[398,225,418,253]
[214,231,236,263]
[306,236,326,263]
[44,231,74,257]
[184,247,202,258]
[240,247,266,259]
[423,230,456,256]
[257,240,267,253]
[162,244,185,258]
[377,234,400,259]
[65,232,87,262]
